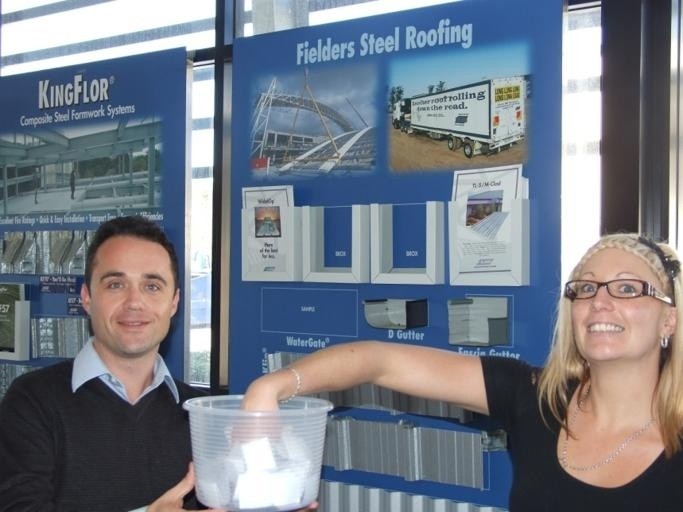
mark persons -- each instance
[0,212,321,512]
[228,233,683,511]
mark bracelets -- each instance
[277,366,301,405]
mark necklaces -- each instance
[562,379,654,472]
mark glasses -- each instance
[565,278,674,307]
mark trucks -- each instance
[392,77,526,159]
